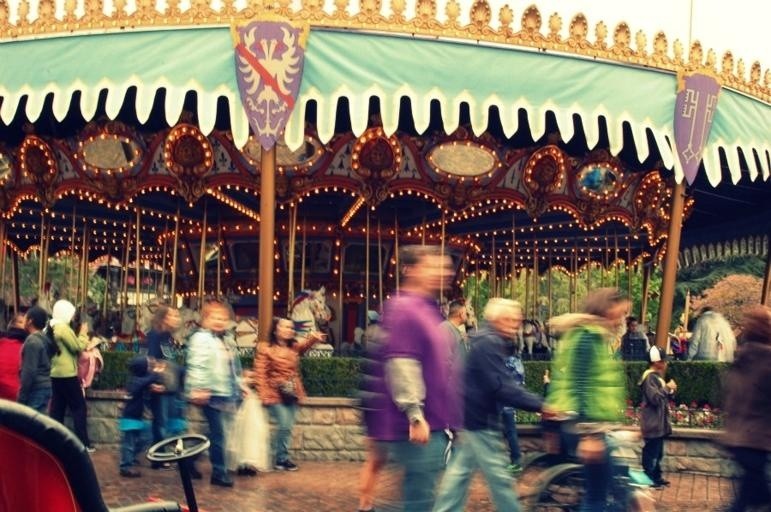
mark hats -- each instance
[646,346,675,362]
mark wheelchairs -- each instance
[512,407,647,512]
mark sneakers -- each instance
[275,460,298,471]
[151,461,171,470]
[211,475,234,487]
[121,468,142,478]
[86,445,96,454]
[651,482,662,488]
[192,469,202,479]
[659,476,669,484]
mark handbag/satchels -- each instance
[278,385,301,405]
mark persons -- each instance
[352,244,771,512]
[1,282,328,488]
